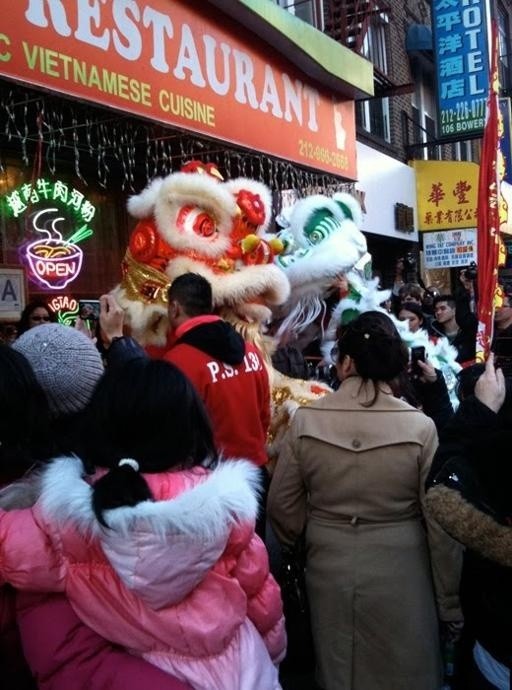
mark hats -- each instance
[13,324,104,416]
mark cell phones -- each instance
[411,346,425,375]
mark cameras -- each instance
[79,299,109,320]
[464,261,478,279]
[403,252,417,272]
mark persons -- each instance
[2,274,511,690]
[102,162,464,486]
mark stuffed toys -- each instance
[102,162,464,484]
